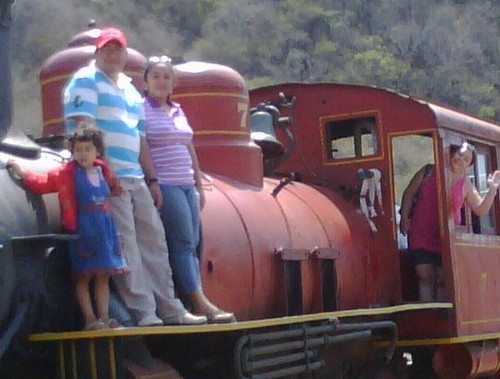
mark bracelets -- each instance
[147,177,158,186]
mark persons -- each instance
[140,54,236,324]
[62,27,207,325]
[398,142,500,305]
[6,121,130,331]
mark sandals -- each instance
[83,316,124,330]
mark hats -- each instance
[95,25,128,50]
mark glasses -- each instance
[148,54,171,63]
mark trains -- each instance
[0,19,500,379]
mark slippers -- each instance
[197,309,238,322]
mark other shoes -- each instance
[135,315,164,325]
[164,312,207,324]
